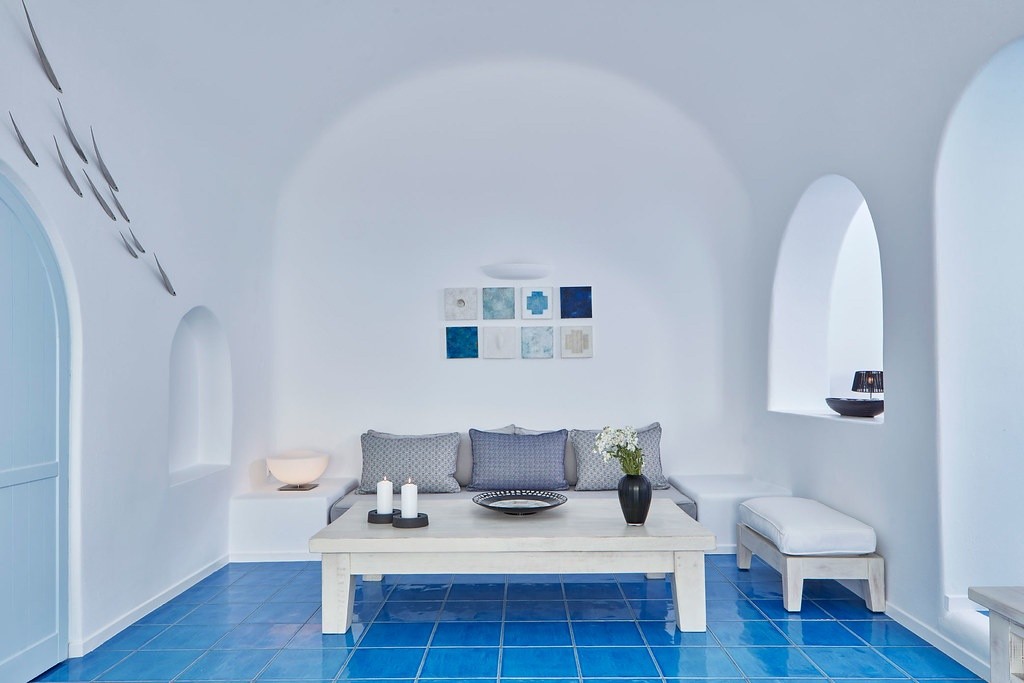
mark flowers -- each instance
[591,425,647,476]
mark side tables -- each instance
[228,476,359,562]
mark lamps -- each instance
[267,447,331,491]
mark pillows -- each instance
[570,422,671,491]
[464,428,571,492]
[354,432,463,495]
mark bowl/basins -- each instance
[826,398,884,418]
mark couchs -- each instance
[330,422,699,524]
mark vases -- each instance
[618,473,653,526]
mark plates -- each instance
[473,490,567,517]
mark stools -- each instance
[737,523,888,614]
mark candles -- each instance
[368,476,401,525]
[392,477,429,528]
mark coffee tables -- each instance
[308,498,717,634]
